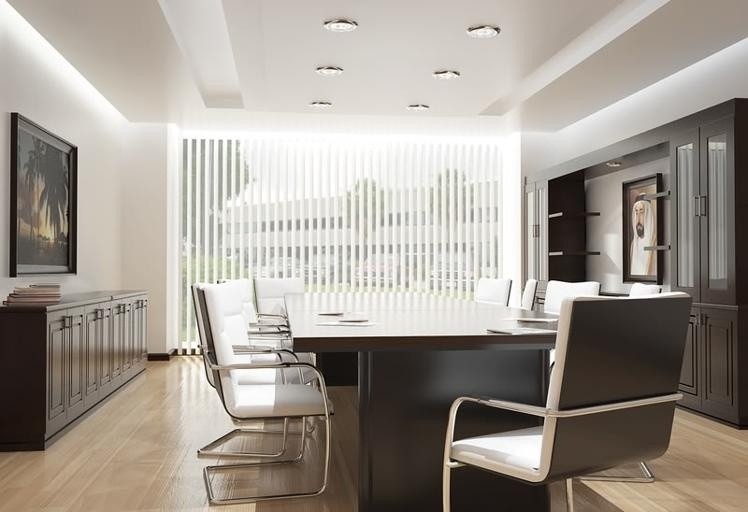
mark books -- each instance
[6,282,63,306]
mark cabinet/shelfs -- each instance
[531,293,546,313]
[0,304,84,452]
[85,300,112,412]
[112,297,132,392]
[523,174,586,291]
[132,294,148,378]
[675,302,744,426]
[669,115,748,306]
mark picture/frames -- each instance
[11,112,78,278]
[622,173,664,285]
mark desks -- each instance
[284,290,560,512]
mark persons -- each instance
[630,193,656,276]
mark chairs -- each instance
[190,277,317,457]
[441,290,692,512]
[544,280,600,316]
[549,283,662,483]
[474,278,512,307]
[191,276,334,506]
[521,278,538,311]
